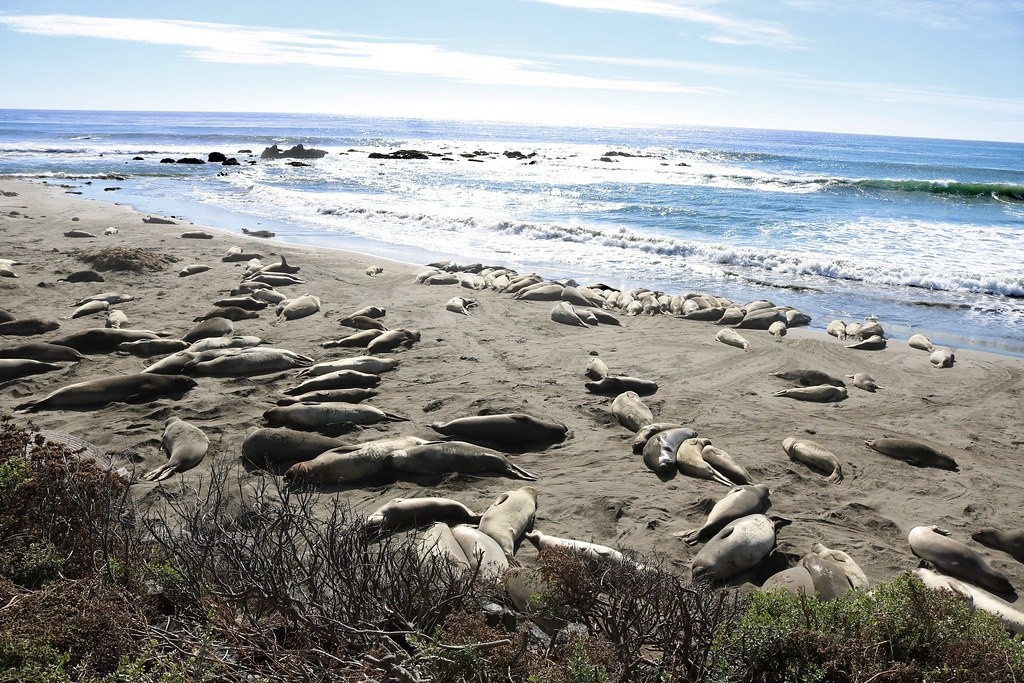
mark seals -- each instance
[0,216,1024,654]
